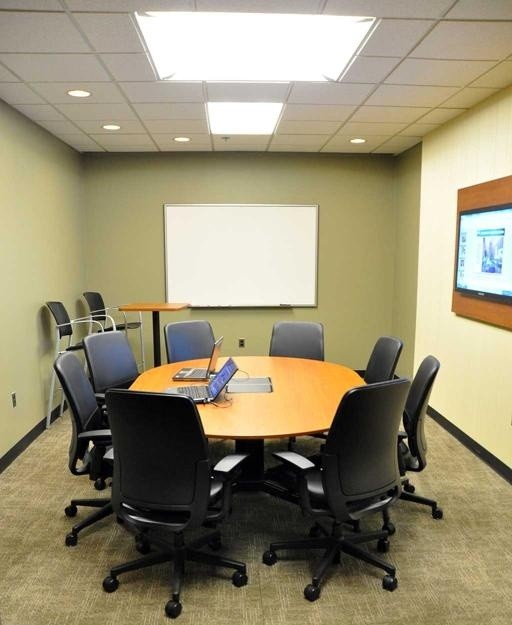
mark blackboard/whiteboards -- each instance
[164,204,319,308]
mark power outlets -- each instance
[10,392,17,409]
[238,338,245,349]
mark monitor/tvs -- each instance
[455,203,512,304]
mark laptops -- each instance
[172,336,225,382]
[167,358,238,404]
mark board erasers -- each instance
[280,303,293,307]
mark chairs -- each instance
[103,388,249,620]
[80,291,142,376]
[163,320,219,365]
[53,351,147,553]
[39,299,116,428]
[263,378,410,602]
[379,351,443,534]
[80,329,141,399]
[359,334,402,386]
[269,320,327,363]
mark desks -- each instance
[126,356,370,506]
